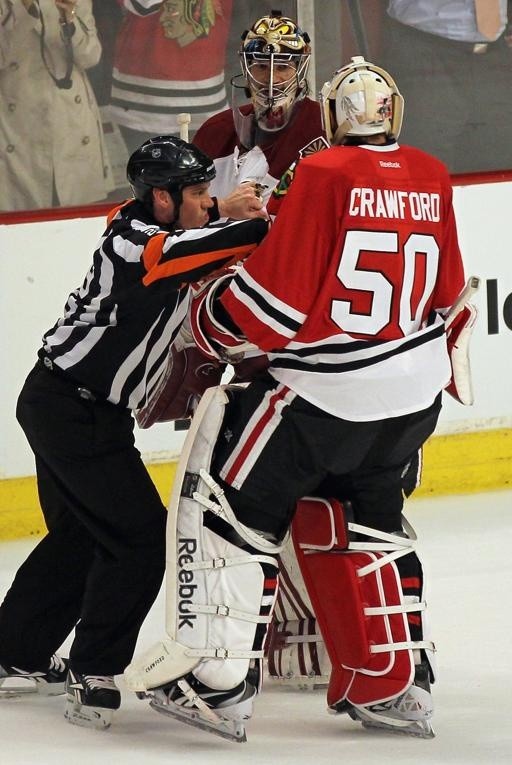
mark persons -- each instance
[144,9,336,423]
[3,134,265,712]
[142,57,478,724]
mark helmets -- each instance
[316,52,408,147]
[228,7,313,152]
[121,134,219,210]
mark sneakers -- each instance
[0,644,71,688]
[150,671,259,722]
[340,671,438,724]
[64,658,122,711]
